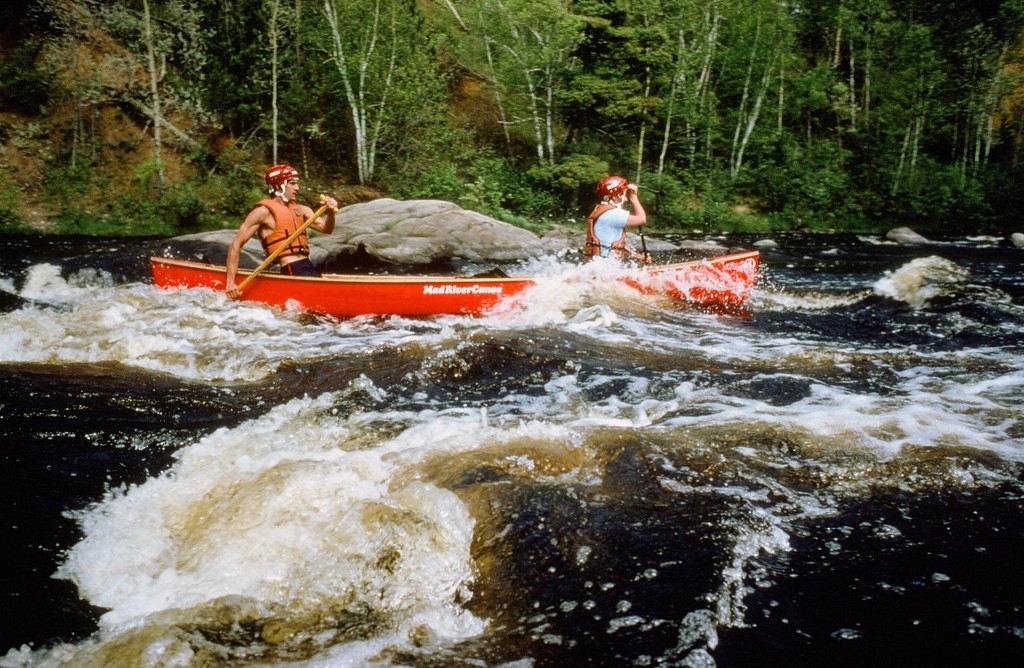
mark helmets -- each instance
[265,165,299,196]
[596,176,630,203]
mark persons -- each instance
[584,176,652,267]
[224,165,338,300]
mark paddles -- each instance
[239,194,339,291]
[638,225,648,266]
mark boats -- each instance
[148,254,538,320]
[454,249,761,315]
